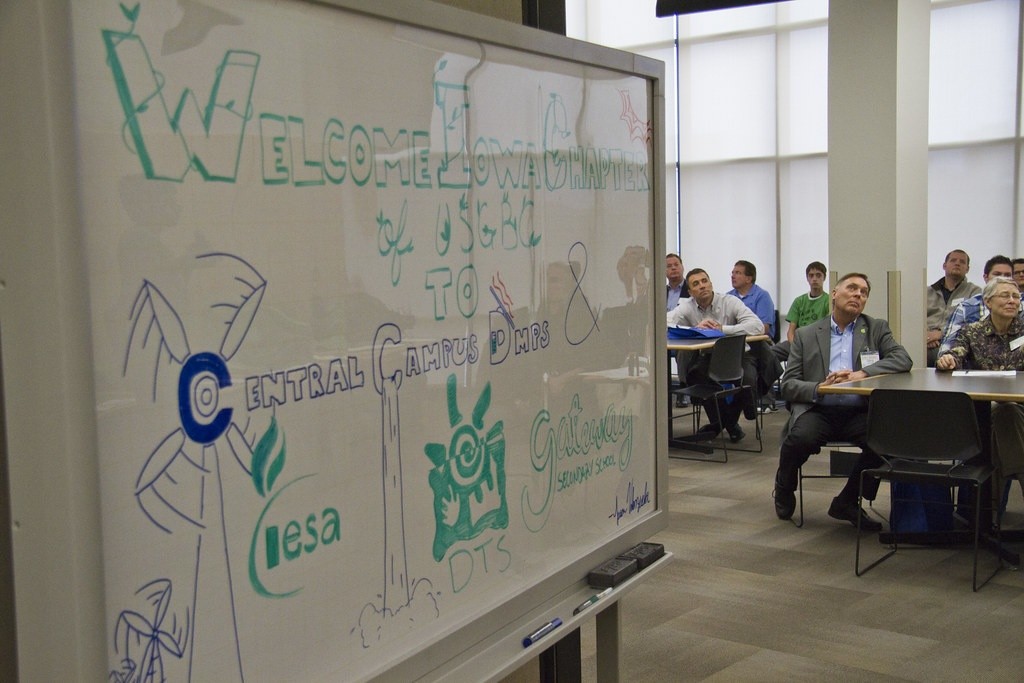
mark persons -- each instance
[726,261,776,339]
[665,253,690,407]
[774,273,914,530]
[1011,258,1024,288]
[937,255,1024,358]
[938,277,1024,522]
[757,262,829,414]
[667,268,766,442]
[927,250,983,368]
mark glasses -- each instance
[993,292,1022,300]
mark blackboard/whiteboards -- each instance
[0,0,674,683]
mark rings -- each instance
[928,336,929,339]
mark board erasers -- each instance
[588,558,638,590]
[616,541,665,572]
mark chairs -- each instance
[666,310,1024,594]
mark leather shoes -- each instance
[726,424,745,443]
[776,468,798,520]
[676,394,688,407]
[828,498,882,531]
[697,423,724,438]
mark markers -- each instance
[522,617,563,648]
[572,588,612,617]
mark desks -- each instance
[666,335,770,455]
[817,367,1024,567]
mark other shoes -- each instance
[757,404,774,414]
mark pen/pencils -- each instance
[965,370,969,374]
